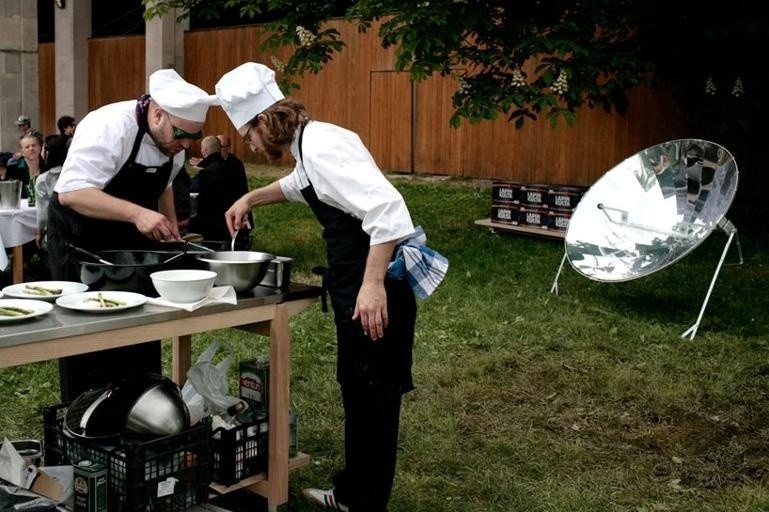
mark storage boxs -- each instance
[490,181,590,232]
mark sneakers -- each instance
[301,488,348,511]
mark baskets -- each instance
[208,412,269,486]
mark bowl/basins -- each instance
[147,268,217,304]
[193,249,277,294]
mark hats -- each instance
[216,62,284,130]
[149,68,218,123]
[14,114,30,125]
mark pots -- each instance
[72,248,186,298]
[162,238,234,254]
[64,374,181,440]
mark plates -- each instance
[1,279,90,300]
[55,290,149,314]
[0,297,53,325]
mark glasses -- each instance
[167,115,203,141]
[241,124,257,145]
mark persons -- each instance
[35,136,67,253]
[216,61,418,511]
[172,164,191,231]
[4,129,47,207]
[215,135,255,229]
[188,136,250,250]
[12,115,35,160]
[44,117,76,170]
[46,67,210,430]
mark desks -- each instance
[1,281,322,512]
[0,198,37,284]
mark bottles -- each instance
[27,172,37,207]
